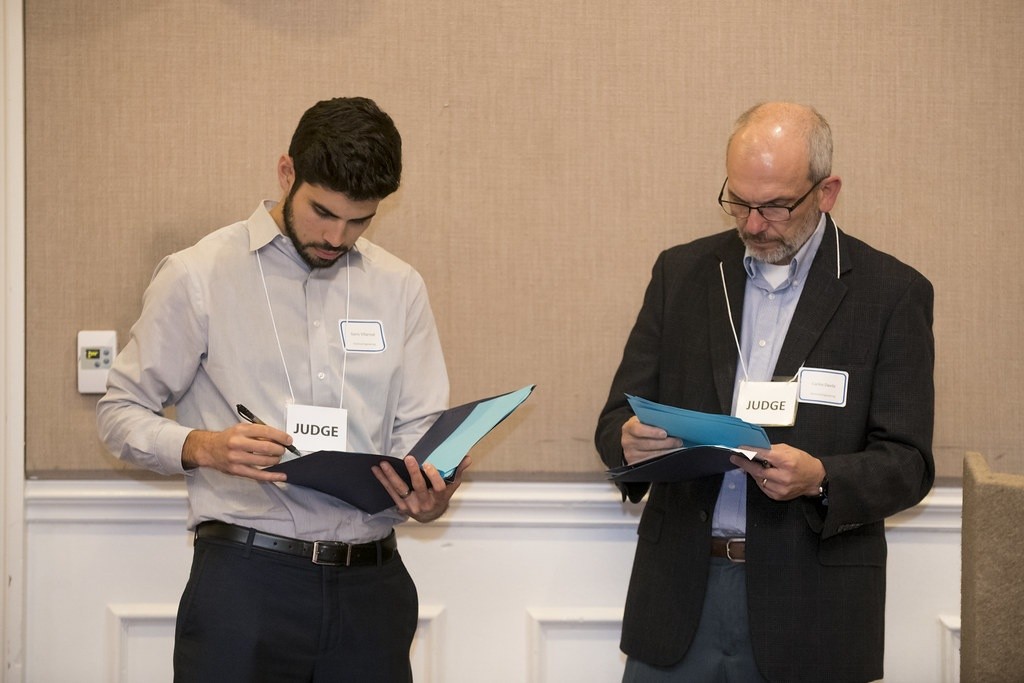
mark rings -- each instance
[399,489,412,499]
[762,478,768,487]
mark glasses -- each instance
[717,175,829,223]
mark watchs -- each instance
[818,475,830,507]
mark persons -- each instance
[593,101,935,682]
[96,97,472,683]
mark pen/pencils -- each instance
[761,459,770,468]
[236,404,302,457]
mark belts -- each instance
[711,538,746,563]
[196,518,398,567]
